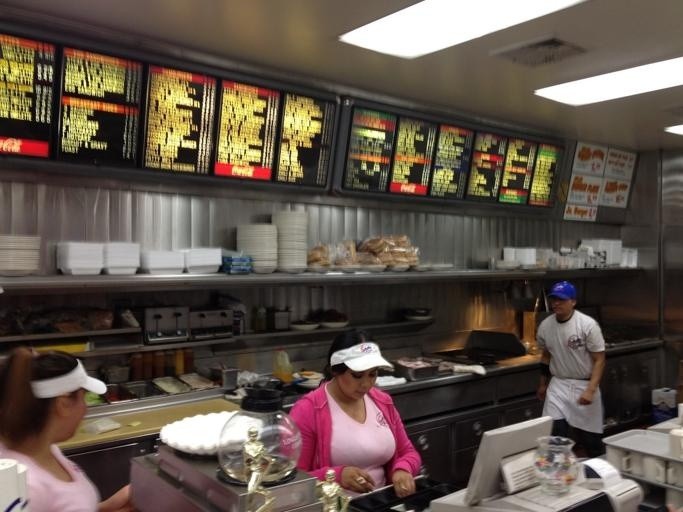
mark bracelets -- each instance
[357,477,367,484]
[538,362,550,377]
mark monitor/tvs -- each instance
[462,415,554,508]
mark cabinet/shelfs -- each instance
[0,266,663,418]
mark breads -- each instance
[309,234,420,267]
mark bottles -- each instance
[272,346,293,385]
[274,305,290,331]
[232,311,243,336]
[256,304,268,332]
[220,384,300,483]
[129,348,194,382]
[533,435,579,495]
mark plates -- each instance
[223,210,309,276]
[1,235,224,276]
[319,322,349,328]
[289,320,320,330]
[312,259,453,273]
[408,314,432,321]
[501,246,539,268]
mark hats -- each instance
[20,357,111,399]
[327,340,397,376]
[546,280,575,302]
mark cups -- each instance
[604,430,682,489]
[664,488,683,512]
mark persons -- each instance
[0,346,130,512]
[537,281,606,458]
[317,470,350,512]
[279,328,421,497]
[242,426,271,508]
